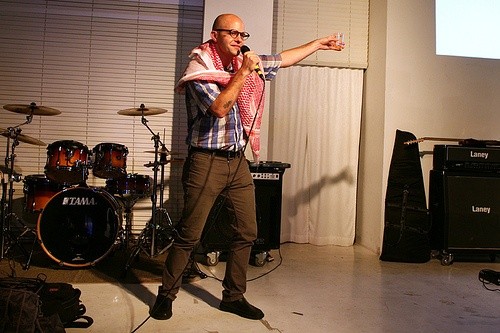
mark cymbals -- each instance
[3,104,61,116]
[118,107,168,116]
[145,150,180,156]
[0,129,46,146]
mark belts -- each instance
[192,147,239,158]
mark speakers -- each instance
[430,169,500,254]
[197,168,282,250]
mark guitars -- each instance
[404,137,500,147]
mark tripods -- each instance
[0,110,38,262]
[125,112,208,278]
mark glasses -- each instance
[215,29,250,41]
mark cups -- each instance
[335,32,344,47]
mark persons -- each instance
[145,15,346,320]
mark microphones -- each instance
[241,45,265,80]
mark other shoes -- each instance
[219,299,264,320]
[151,294,173,320]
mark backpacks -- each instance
[32,282,93,328]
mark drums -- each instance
[92,142,129,179]
[24,174,72,214]
[106,173,150,198]
[45,140,89,183]
[37,186,122,268]
